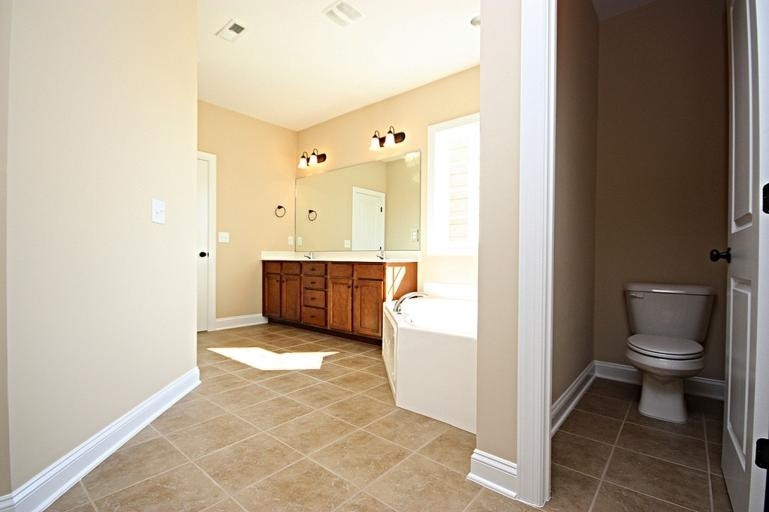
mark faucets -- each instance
[393,292,428,312]
[377,244,386,261]
[303,252,312,258]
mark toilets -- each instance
[624,281,717,426]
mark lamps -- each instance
[369,125,405,151]
[298,148,326,169]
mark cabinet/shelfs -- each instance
[262,261,417,346]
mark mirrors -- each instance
[295,151,421,252]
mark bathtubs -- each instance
[383,297,478,433]
